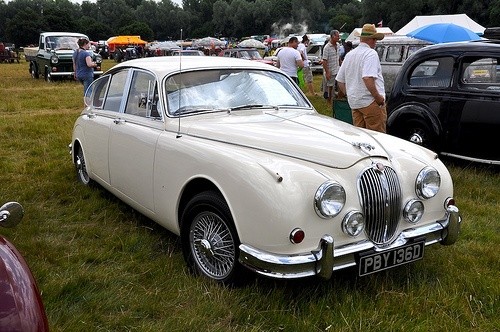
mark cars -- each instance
[0,202,49,332]
[0,42,19,64]
[386,25,500,166]
[68,56,463,288]
[89,33,500,102]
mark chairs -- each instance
[332,97,352,123]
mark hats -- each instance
[356,24,385,40]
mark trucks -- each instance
[23,31,104,83]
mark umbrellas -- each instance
[148,41,180,51]
[238,39,266,49]
[191,36,224,50]
[405,23,481,44]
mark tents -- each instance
[344,27,393,43]
[391,13,486,36]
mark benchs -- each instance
[412,76,450,86]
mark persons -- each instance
[334,24,387,134]
[297,35,318,96]
[319,30,354,102]
[71,38,97,107]
[277,37,304,87]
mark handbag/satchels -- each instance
[297,67,304,87]
